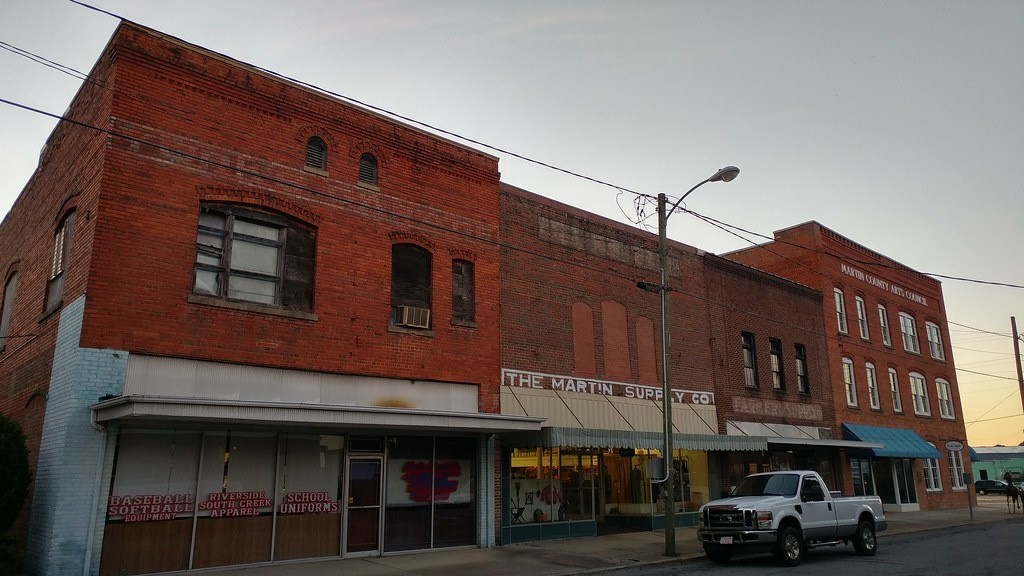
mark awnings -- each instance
[841,422,943,458]
[515,426,769,452]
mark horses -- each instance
[1003,470,1024,514]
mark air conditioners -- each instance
[393,305,430,328]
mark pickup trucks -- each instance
[695,469,888,567]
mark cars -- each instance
[1013,481,1024,491]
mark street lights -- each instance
[658,165,743,556]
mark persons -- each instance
[603,465,613,505]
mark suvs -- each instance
[975,480,1017,496]
